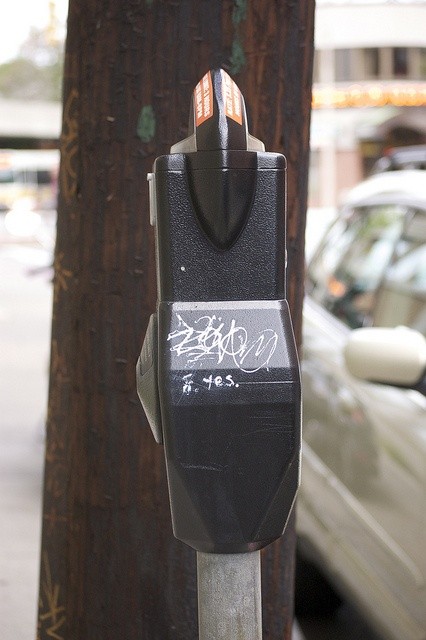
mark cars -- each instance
[300,165,426,639]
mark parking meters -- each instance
[133,67,302,637]
